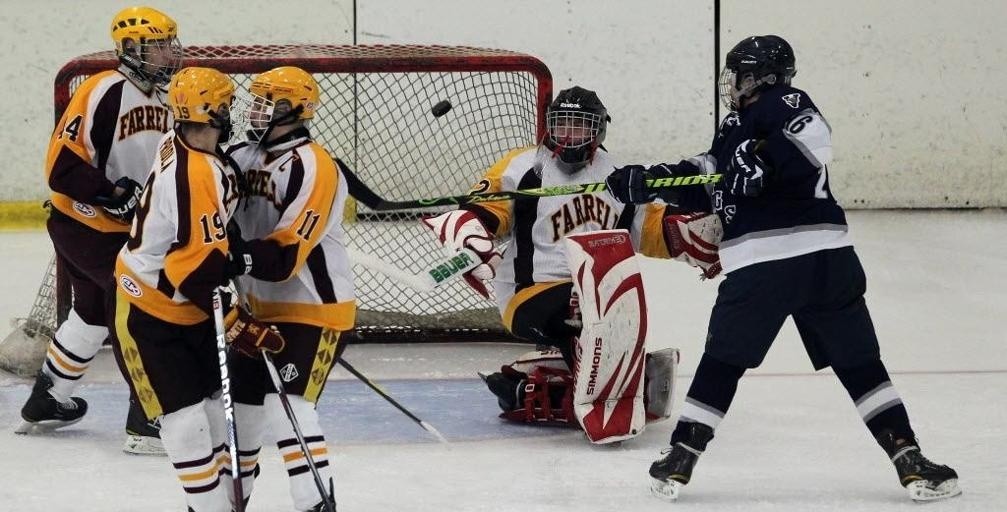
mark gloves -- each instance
[602,162,671,208]
[718,135,774,201]
[104,175,145,228]
[229,238,275,281]
[419,204,505,300]
[222,303,295,367]
[659,204,726,282]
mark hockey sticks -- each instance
[346,246,482,294]
[333,155,723,212]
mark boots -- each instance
[123,387,164,439]
[647,419,717,487]
[484,363,531,413]
[19,366,90,423]
[872,425,958,488]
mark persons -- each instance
[214,64,356,512]
[456,83,725,420]
[106,67,249,512]
[603,34,962,487]
[20,5,183,439]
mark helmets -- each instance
[721,32,799,100]
[105,3,180,60]
[247,64,321,125]
[544,84,612,146]
[165,64,238,127]
